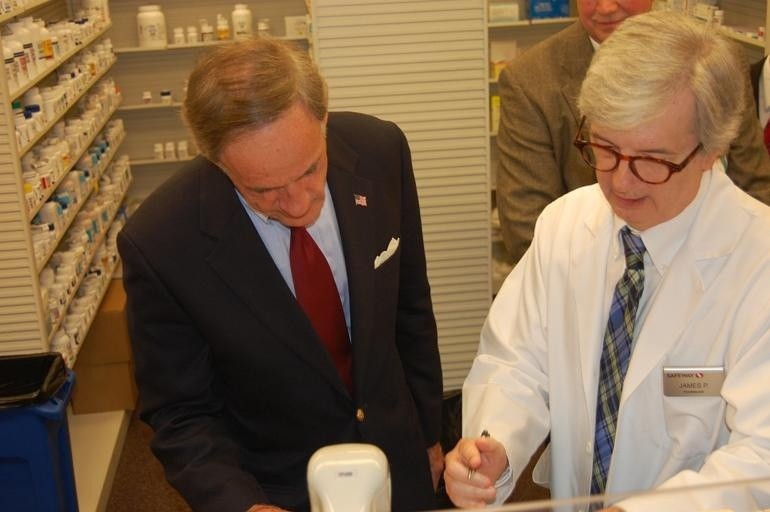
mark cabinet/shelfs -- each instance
[111,0,314,283]
[0,1,133,371]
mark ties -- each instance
[354,227,357,229]
[290,228,354,411]
[590,226,647,510]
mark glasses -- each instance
[572,116,703,184]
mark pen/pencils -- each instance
[468,431,490,480]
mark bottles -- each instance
[141,91,154,104]
[160,92,174,105]
[154,141,189,161]
[715,9,724,31]
[137,1,271,46]
[2,0,134,369]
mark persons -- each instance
[115,35,449,512]
[440,7,769,512]
